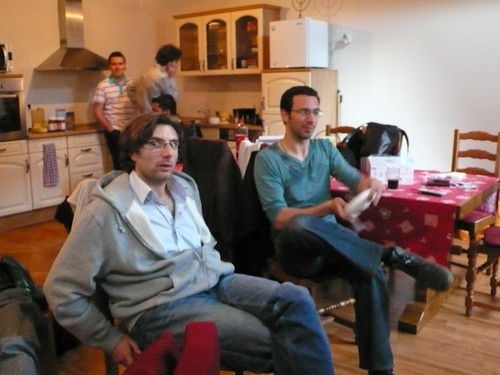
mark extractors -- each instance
[34,0,127,72]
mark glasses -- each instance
[290,108,323,118]
[145,137,181,150]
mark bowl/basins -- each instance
[208,116,221,125]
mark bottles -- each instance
[55,108,66,117]
[240,58,247,68]
[48,117,57,132]
[66,112,76,130]
[56,116,68,132]
[235,118,249,160]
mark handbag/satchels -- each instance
[343,121,410,159]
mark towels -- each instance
[42,143,59,187]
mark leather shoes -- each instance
[384,245,453,292]
[0,256,47,313]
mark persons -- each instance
[93,50,136,170]
[126,43,183,115]
[152,94,182,122]
[254,86,437,375]
[42,111,335,374]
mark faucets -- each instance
[197,109,210,120]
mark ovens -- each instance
[0,90,30,142]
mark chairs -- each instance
[249,150,383,320]
[362,126,401,157]
[449,129,500,289]
[56,194,244,375]
[465,226,500,317]
[326,124,354,152]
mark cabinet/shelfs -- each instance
[230,3,281,75]
[67,133,114,193]
[262,70,338,137]
[28,136,71,210]
[0,140,32,218]
[173,8,230,76]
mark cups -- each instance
[386,167,401,190]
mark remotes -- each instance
[418,187,448,197]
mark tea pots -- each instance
[0,44,9,73]
